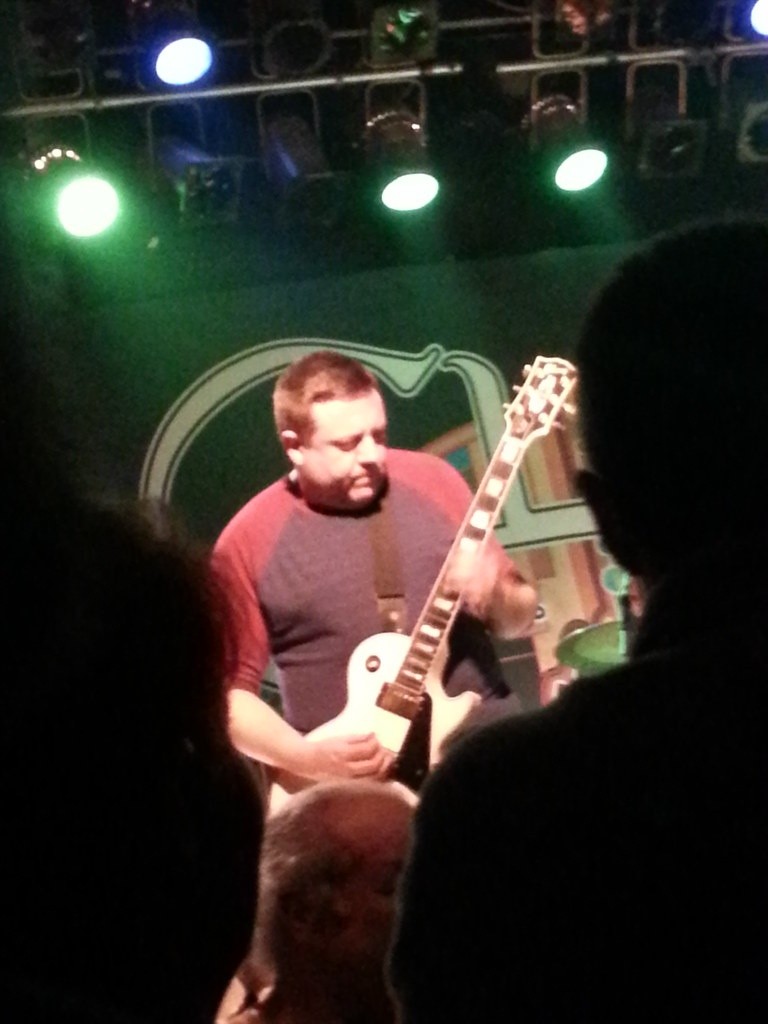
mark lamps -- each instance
[2,1,767,245]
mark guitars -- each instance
[216,353,580,818]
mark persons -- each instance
[205,348,540,793]
[386,208,768,1024]
[0,452,272,1024]
[224,777,422,1024]
